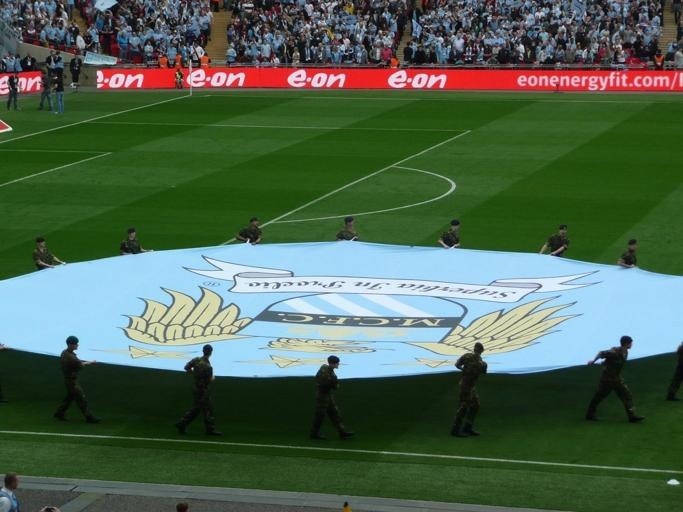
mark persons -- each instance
[236,218,262,246]
[176,502,189,512]
[0,471,19,512]
[0,0,214,111]
[54,336,102,424]
[336,217,359,241]
[311,356,356,440]
[120,228,147,256]
[176,345,223,435]
[586,336,645,424]
[33,237,65,270]
[439,220,461,248]
[539,225,569,257]
[224,0,683,70]
[451,343,487,437]
[618,239,637,268]
[666,342,683,402]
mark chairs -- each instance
[2,1,682,72]
